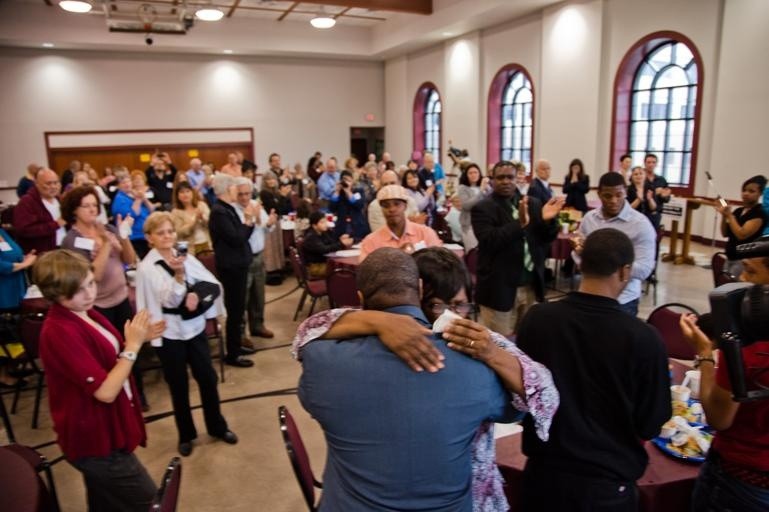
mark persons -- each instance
[1,150,769,511]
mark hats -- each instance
[375,184,410,204]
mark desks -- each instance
[322,244,465,276]
[493,403,702,510]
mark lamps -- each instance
[57,1,337,33]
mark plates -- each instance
[678,397,716,429]
[652,429,717,462]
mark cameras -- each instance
[340,180,349,188]
[177,241,188,258]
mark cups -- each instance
[671,385,690,405]
[686,369,703,399]
[562,222,570,235]
[288,213,297,222]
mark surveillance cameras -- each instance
[144,35,153,44]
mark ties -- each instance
[511,204,535,272]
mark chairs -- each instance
[0,304,46,429]
[2,444,62,510]
[287,245,330,321]
[149,455,183,510]
[327,265,361,312]
[646,302,704,364]
[637,224,664,306]
[204,319,226,383]
[194,250,217,282]
[551,238,578,293]
[276,403,323,510]
[711,250,732,290]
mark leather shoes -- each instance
[221,429,237,444]
[226,325,274,367]
[178,438,191,456]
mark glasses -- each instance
[429,302,477,314]
